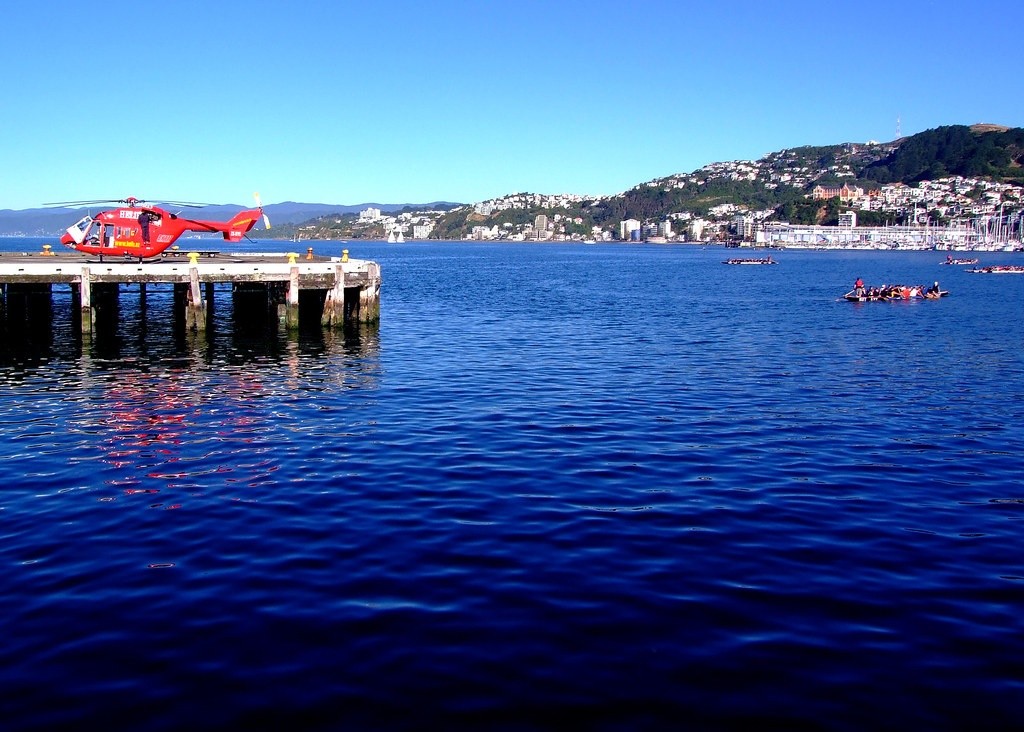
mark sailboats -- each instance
[783,204,1024,253]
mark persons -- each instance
[138,209,152,245]
[854,277,940,299]
[983,264,1023,271]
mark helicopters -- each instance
[42,190,274,264]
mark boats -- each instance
[938,258,978,265]
[842,290,950,302]
[721,254,779,265]
[963,265,1024,274]
